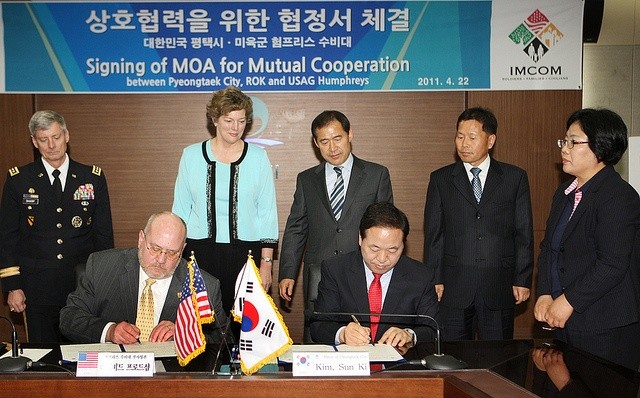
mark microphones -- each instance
[314,311,460,370]
[0,315,33,372]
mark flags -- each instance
[230,255,294,376]
[174,255,215,367]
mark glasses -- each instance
[144,234,183,261]
[557,139,589,149]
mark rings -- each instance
[11,308,14,311]
[166,326,170,330]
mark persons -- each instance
[278,110,394,306]
[308,200,439,347]
[59,210,235,344]
[394,343,410,355]
[171,85,279,343]
[532,346,589,397]
[422,106,534,341]
[532,108,639,369]
[1,110,114,344]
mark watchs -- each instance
[261,256,272,262]
[406,329,415,341]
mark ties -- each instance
[471,168,483,204]
[368,272,382,342]
[52,169,62,199]
[329,167,345,222]
[135,278,156,342]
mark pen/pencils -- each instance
[125,319,141,344]
[351,314,375,346]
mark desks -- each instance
[0,339,639,398]
